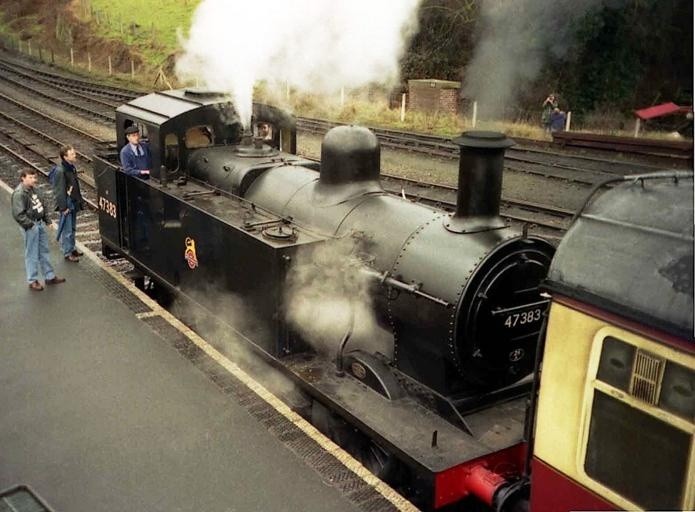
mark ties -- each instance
[137,146,141,156]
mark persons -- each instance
[117,126,154,175]
[8,166,65,291]
[538,93,559,127]
[550,108,568,133]
[46,140,88,264]
[255,122,274,141]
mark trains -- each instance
[90,85,695,511]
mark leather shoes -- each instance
[72,250,83,256]
[65,254,79,262]
[31,281,43,290]
[45,277,65,285]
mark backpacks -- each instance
[48,167,57,186]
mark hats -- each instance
[125,127,139,133]
